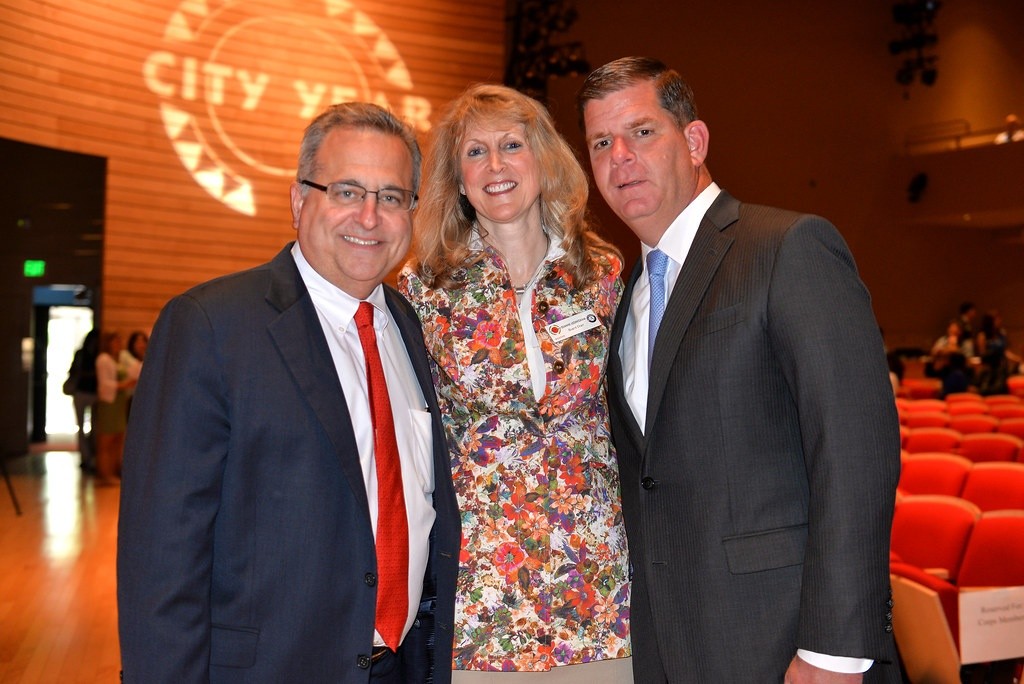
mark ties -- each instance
[646,250,669,384]
[353,300,412,653]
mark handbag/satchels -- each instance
[63,377,77,394]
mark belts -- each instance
[371,647,390,665]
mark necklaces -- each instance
[513,234,550,295]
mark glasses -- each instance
[299,178,419,213]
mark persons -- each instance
[581,55,901,684]
[397,84,629,684]
[925,303,1024,402]
[67,103,462,684]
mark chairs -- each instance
[894,376,1024,684]
